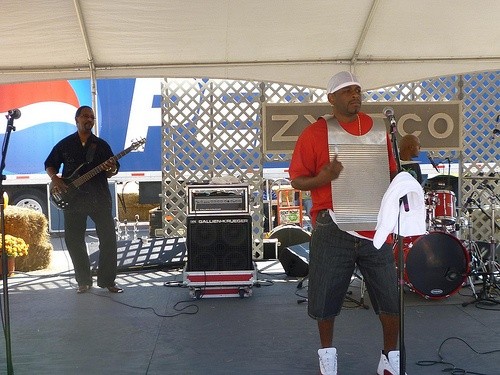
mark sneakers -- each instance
[376,349,400,375]
[318,347,338,375]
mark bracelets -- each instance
[112,168,117,174]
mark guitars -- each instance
[50,136,147,209]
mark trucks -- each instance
[0,71,500,242]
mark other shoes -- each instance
[77,281,93,293]
[97,281,124,293]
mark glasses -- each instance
[77,114,94,121]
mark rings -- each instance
[109,167,111,171]
[53,186,55,190]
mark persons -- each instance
[289,71,407,375]
[44,106,124,294]
[258,180,278,232]
[399,135,422,185]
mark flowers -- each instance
[0,233,30,257]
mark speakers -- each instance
[186,214,252,271]
[278,241,311,277]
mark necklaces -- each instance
[82,142,85,146]
[356,114,361,136]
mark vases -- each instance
[0,255,16,274]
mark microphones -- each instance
[461,192,474,212]
[448,271,456,279]
[382,106,397,133]
[426,154,440,173]
[7,109,21,119]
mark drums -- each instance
[392,231,470,300]
[427,190,457,226]
